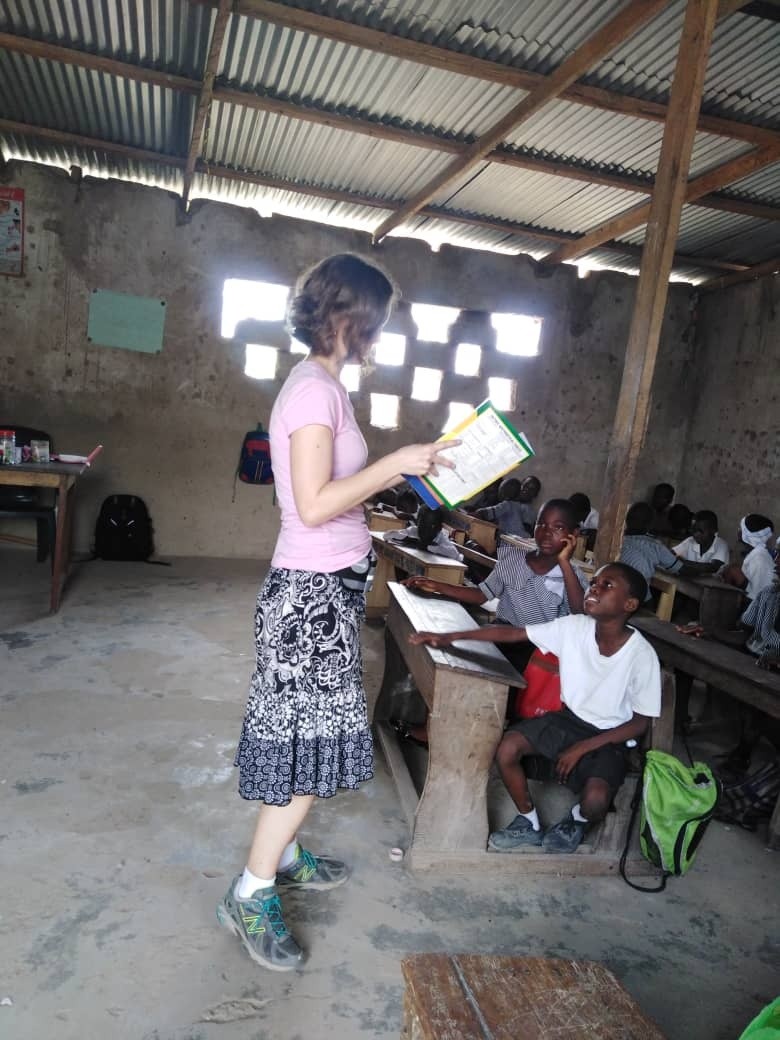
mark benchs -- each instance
[464,577,502,624]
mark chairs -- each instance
[0,425,61,564]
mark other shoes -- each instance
[725,729,760,777]
[407,724,427,748]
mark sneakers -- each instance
[541,806,590,852]
[487,815,545,851]
[215,875,306,972]
[277,839,348,891]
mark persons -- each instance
[718,514,780,770]
[407,561,660,852]
[566,493,598,529]
[373,477,424,528]
[461,475,541,538]
[396,499,590,750]
[382,500,459,624]
[651,482,729,587]
[217,251,461,972]
[618,501,709,614]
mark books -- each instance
[399,399,535,510]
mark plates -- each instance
[60,455,86,463]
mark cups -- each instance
[0,430,16,465]
[31,440,50,463]
[15,447,22,464]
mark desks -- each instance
[361,498,780,875]
[400,952,665,1040]
[0,458,89,613]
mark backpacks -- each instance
[621,715,722,895]
[237,422,275,485]
[712,760,780,832]
[91,494,153,562]
